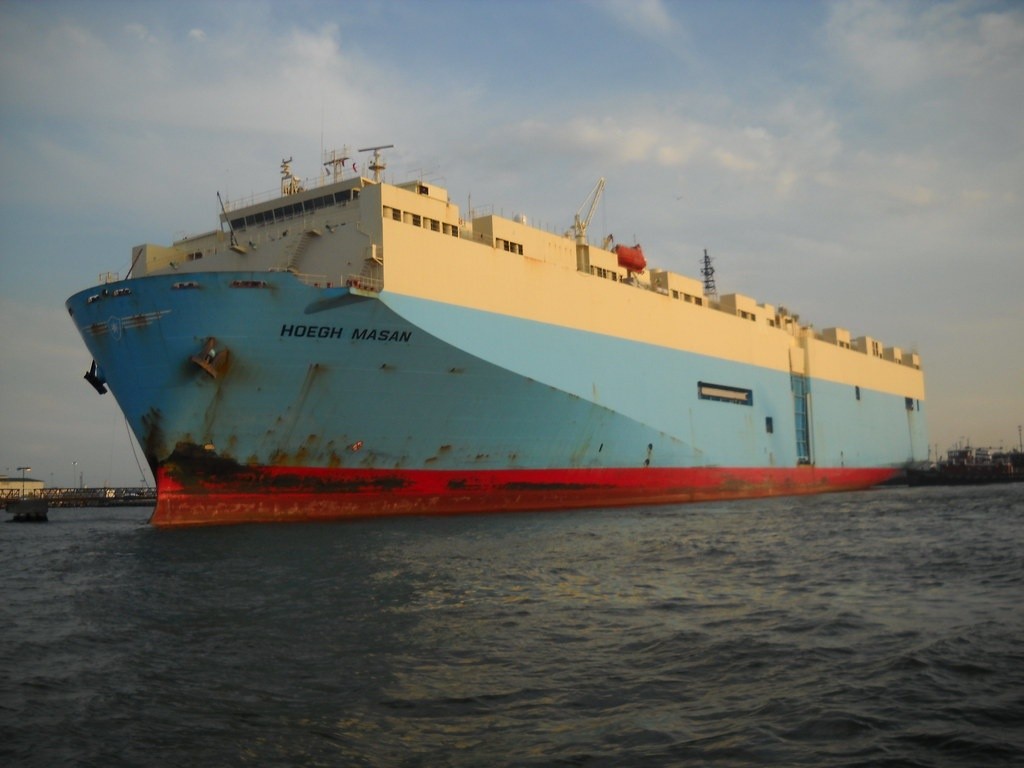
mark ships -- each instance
[63,142,928,526]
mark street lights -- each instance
[71,461,77,489]
[17,467,31,500]
